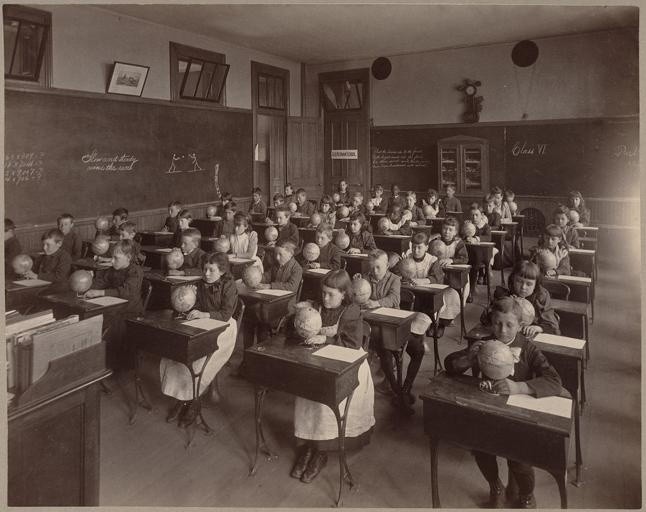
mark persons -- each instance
[159,180,591,509]
[4,207,147,370]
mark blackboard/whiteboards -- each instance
[370,115,640,203]
[5,81,260,233]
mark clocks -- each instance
[458,80,483,123]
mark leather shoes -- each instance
[291,447,315,478]
[519,492,535,509]
[168,401,184,423]
[489,487,506,508]
[179,399,200,427]
[301,451,327,483]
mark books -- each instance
[5,309,104,402]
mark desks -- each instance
[355,304,418,416]
[463,322,587,489]
[416,368,584,508]
[398,281,451,376]
[123,309,232,450]
[9,206,527,340]
[534,207,598,364]
[244,341,372,507]
[9,341,115,507]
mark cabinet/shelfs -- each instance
[438,136,489,196]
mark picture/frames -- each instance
[106,61,150,97]
